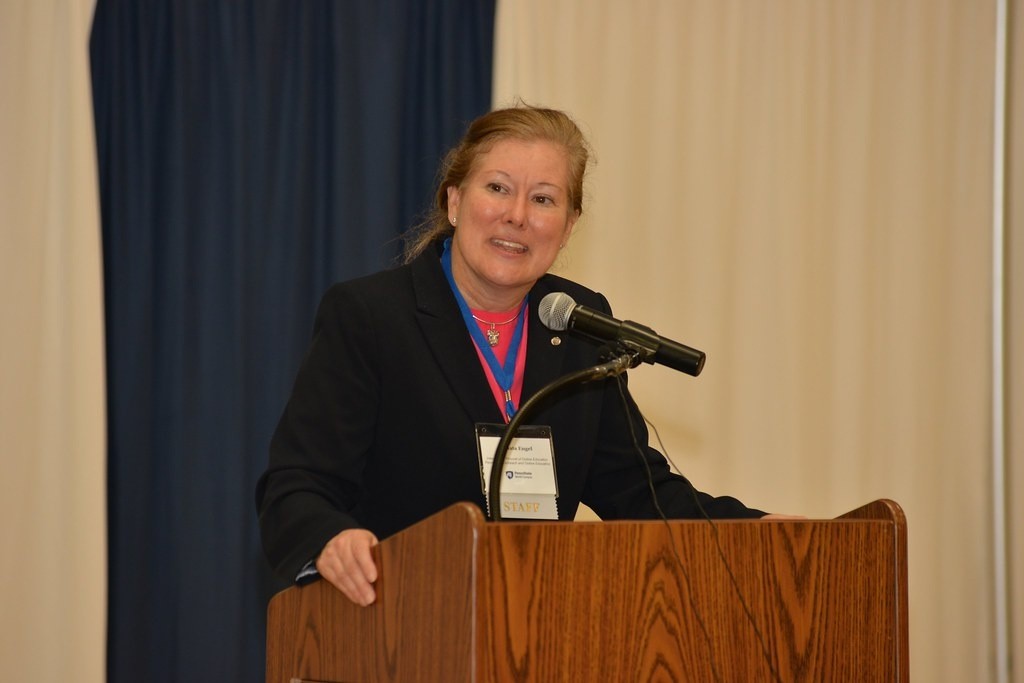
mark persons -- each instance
[254,109,813,607]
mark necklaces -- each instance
[472,312,519,346]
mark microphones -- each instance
[538,292,706,377]
